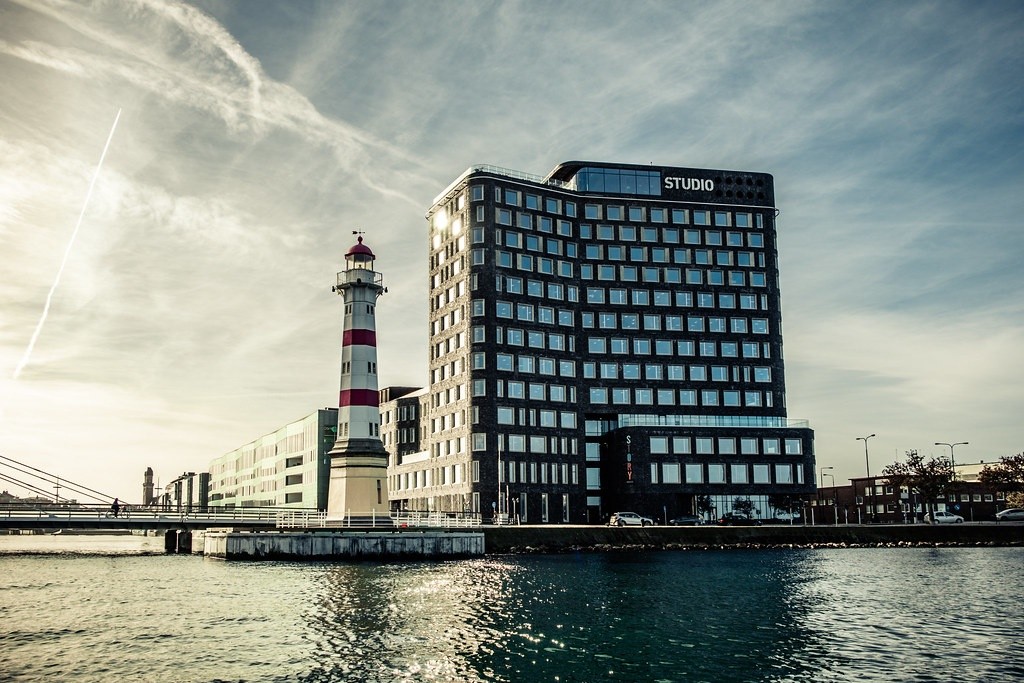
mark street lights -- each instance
[856,434,876,523]
[935,442,969,517]
[820,466,833,489]
[823,473,835,486]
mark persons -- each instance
[111,498,122,518]
[613,517,623,527]
[928,505,936,526]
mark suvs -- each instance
[609,512,654,526]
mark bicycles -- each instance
[106,504,130,519]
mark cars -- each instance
[719,514,762,526]
[924,510,965,524]
[995,508,1024,522]
[669,513,705,526]
